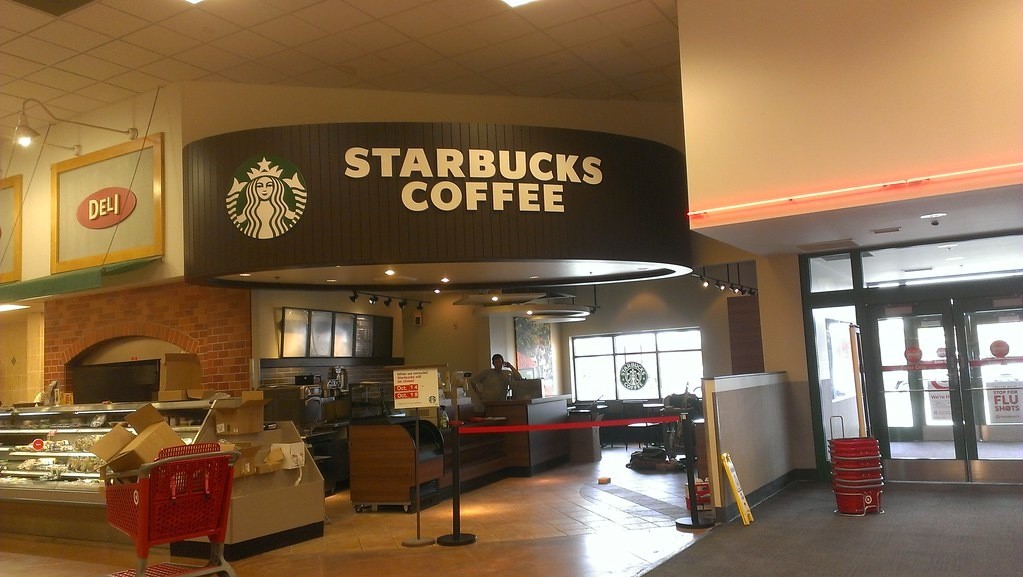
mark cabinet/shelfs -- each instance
[440,419,510,501]
[1,400,215,548]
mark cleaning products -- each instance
[440,405,449,429]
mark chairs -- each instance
[569,399,664,450]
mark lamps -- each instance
[349,290,431,310]
[691,262,758,297]
[12,98,138,147]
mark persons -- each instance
[470,354,522,401]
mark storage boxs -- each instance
[89,403,187,484]
[188,390,215,400]
[158,390,187,402]
[220,441,305,478]
[565,412,604,462]
[216,390,272,434]
[158,353,202,390]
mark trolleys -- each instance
[104,443,242,577]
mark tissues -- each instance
[271,442,305,486]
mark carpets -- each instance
[639,479,1023,577]
